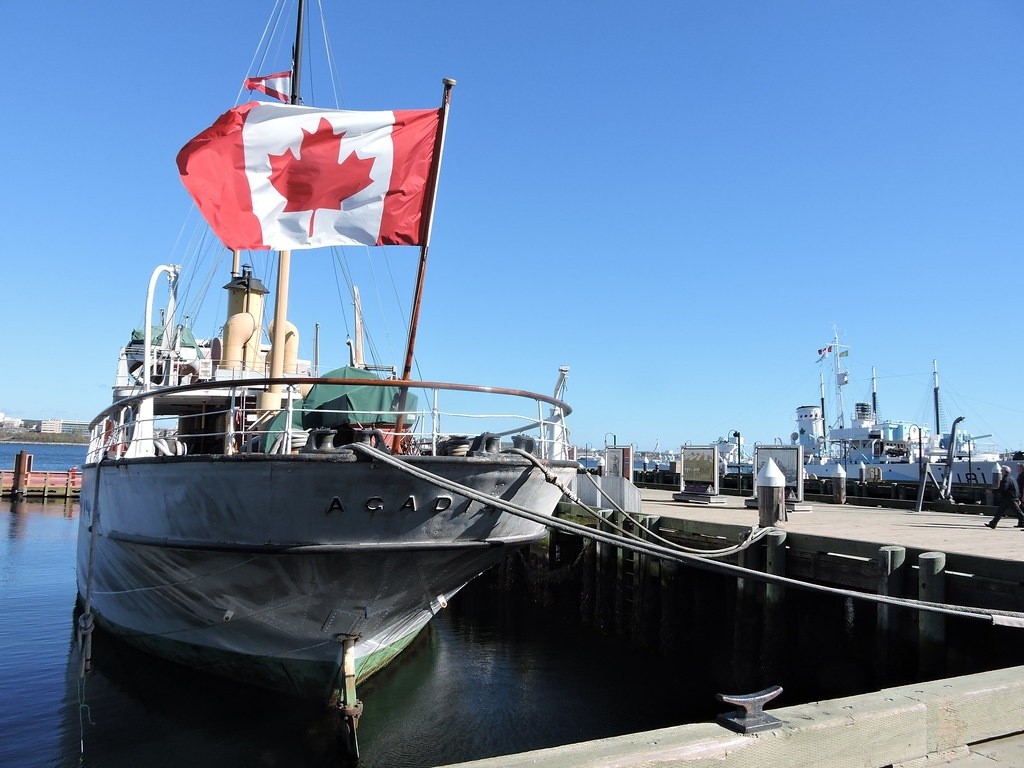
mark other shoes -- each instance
[1014,525,1024,528]
[1021,529,1024,531]
[985,523,995,529]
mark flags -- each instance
[174,101,440,249]
[818,346,832,354]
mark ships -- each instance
[719,323,1023,510]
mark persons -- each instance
[985,462,1024,529]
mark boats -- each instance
[69,0,575,761]
